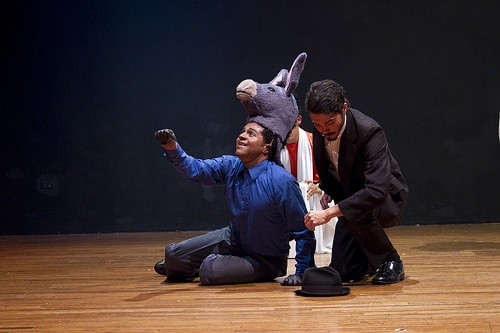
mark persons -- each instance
[279,114,338,259]
[304,79,408,285]
[154,122,316,287]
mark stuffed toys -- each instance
[236,53,307,169]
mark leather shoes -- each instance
[371,260,405,285]
[154,259,166,275]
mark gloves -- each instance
[154,129,177,150]
[282,275,302,285]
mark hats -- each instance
[235,52,307,142]
[294,266,351,296]
